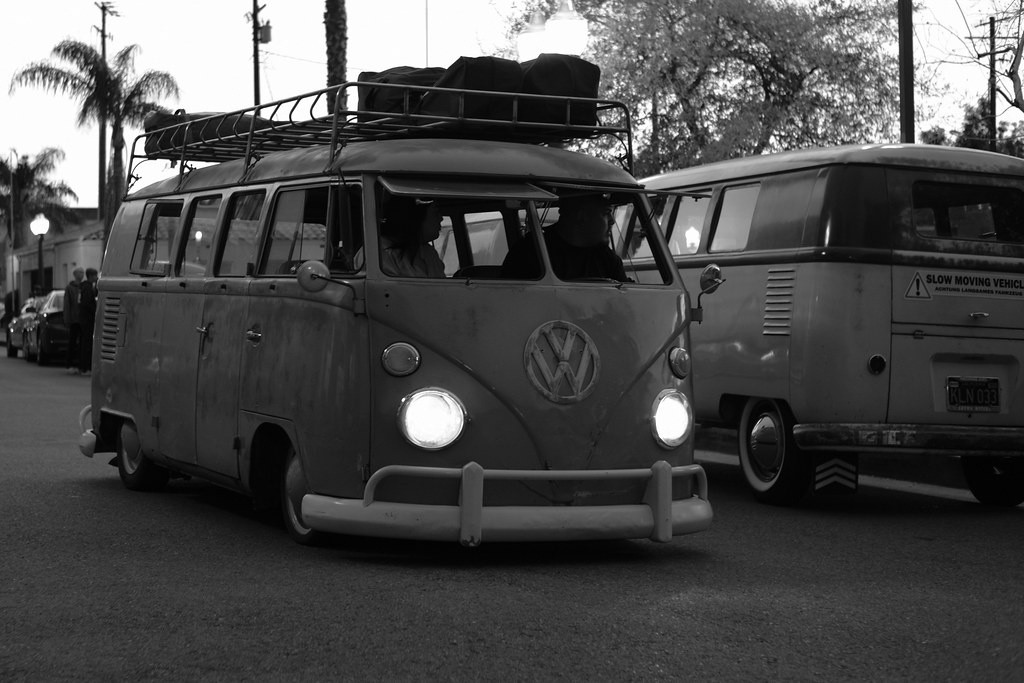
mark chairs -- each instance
[275,259,324,274]
[453,265,506,279]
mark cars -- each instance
[4,286,97,367]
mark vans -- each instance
[74,86,727,549]
[433,203,562,278]
[606,138,1024,514]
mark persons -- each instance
[502,193,635,283]
[63,267,99,375]
[353,193,447,278]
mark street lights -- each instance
[27,211,51,295]
